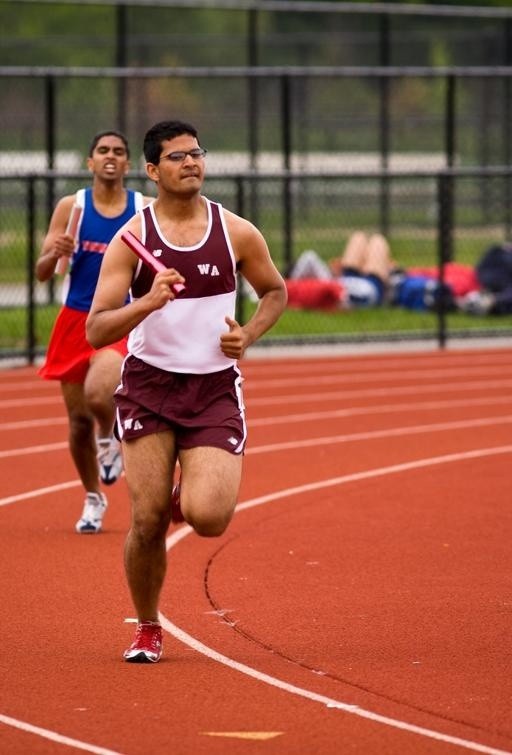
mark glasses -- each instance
[160,147,209,162]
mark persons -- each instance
[291,229,398,310]
[87,122,288,664]
[36,130,157,532]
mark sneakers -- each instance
[74,490,108,535]
[121,618,164,665]
[95,430,124,486]
